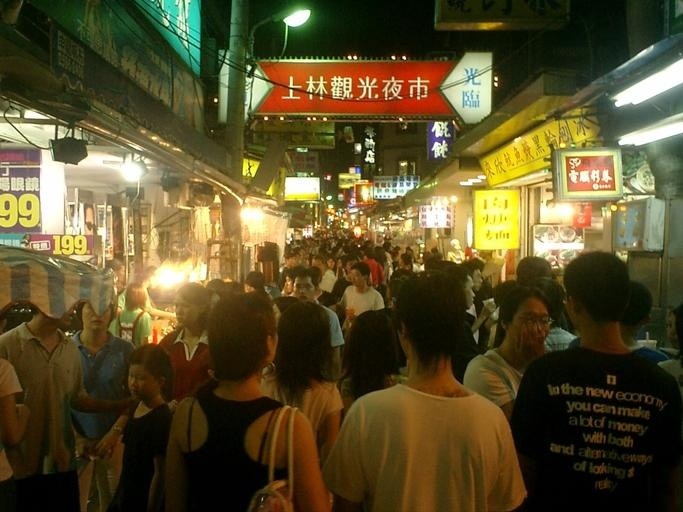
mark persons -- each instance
[107,258,180,349]
[0,303,140,511]
[159,225,681,511]
[69,294,138,511]
[0,355,32,510]
[108,344,174,510]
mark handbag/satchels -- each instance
[248,477,334,512]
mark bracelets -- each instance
[114,422,123,433]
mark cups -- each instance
[638,340,657,350]
[483,298,495,313]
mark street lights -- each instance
[219,7,312,274]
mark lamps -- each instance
[49,123,88,165]
[121,152,148,183]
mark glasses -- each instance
[513,314,554,326]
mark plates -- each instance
[534,227,576,268]
